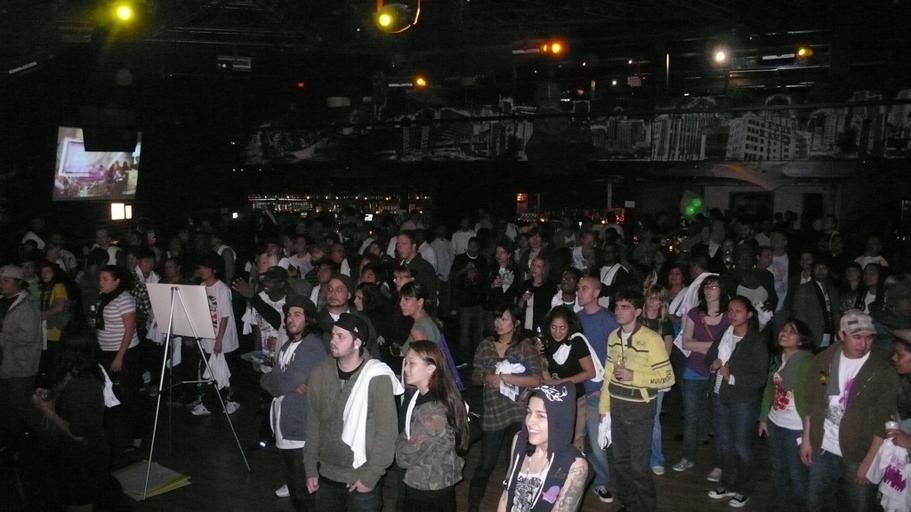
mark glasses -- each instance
[704,285,720,290]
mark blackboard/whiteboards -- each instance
[145,283,216,339]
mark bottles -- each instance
[886,410,899,440]
[615,352,623,380]
[32,392,53,402]
[90,305,97,327]
[297,264,301,279]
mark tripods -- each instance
[143,286,251,501]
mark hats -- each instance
[260,266,287,281]
[335,313,369,347]
[282,295,315,312]
[840,313,876,336]
[0,264,30,288]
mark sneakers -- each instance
[275,485,291,497]
[652,457,693,475]
[593,486,613,503]
[707,468,750,508]
[140,384,240,416]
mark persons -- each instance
[0,207,911,511]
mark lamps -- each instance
[795,46,814,66]
[375,1,422,33]
